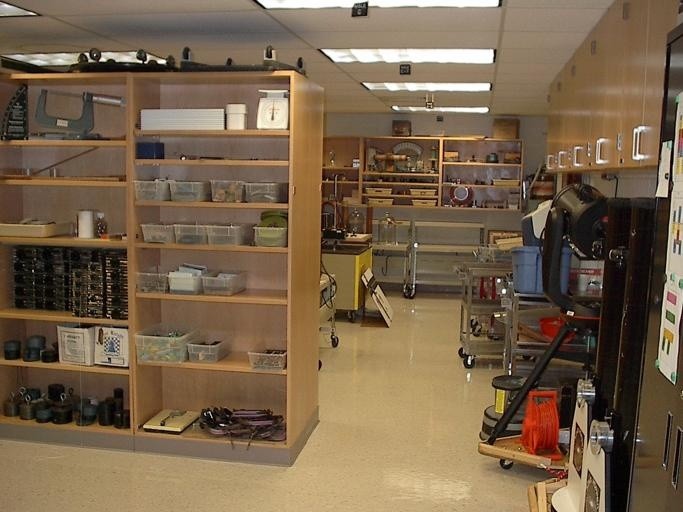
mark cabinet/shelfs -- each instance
[0,69,325,467]
[323,134,524,213]
[544,1,683,174]
[627,23,683,512]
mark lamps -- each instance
[0,50,167,68]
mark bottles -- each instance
[376,212,397,246]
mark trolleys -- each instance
[451,263,513,368]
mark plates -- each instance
[258,211,287,247]
[450,186,474,204]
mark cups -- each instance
[78,210,94,239]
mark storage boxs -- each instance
[174,223,206,244]
[244,182,287,203]
[253,226,288,247]
[169,180,210,202]
[130,179,168,201]
[135,266,178,293]
[168,265,213,295]
[206,223,254,245]
[491,117,520,140]
[93,326,128,368]
[186,333,232,362]
[210,180,244,203]
[508,246,570,295]
[201,269,248,296]
[139,221,172,243]
[54,322,93,366]
[134,323,198,362]
[247,336,286,370]
